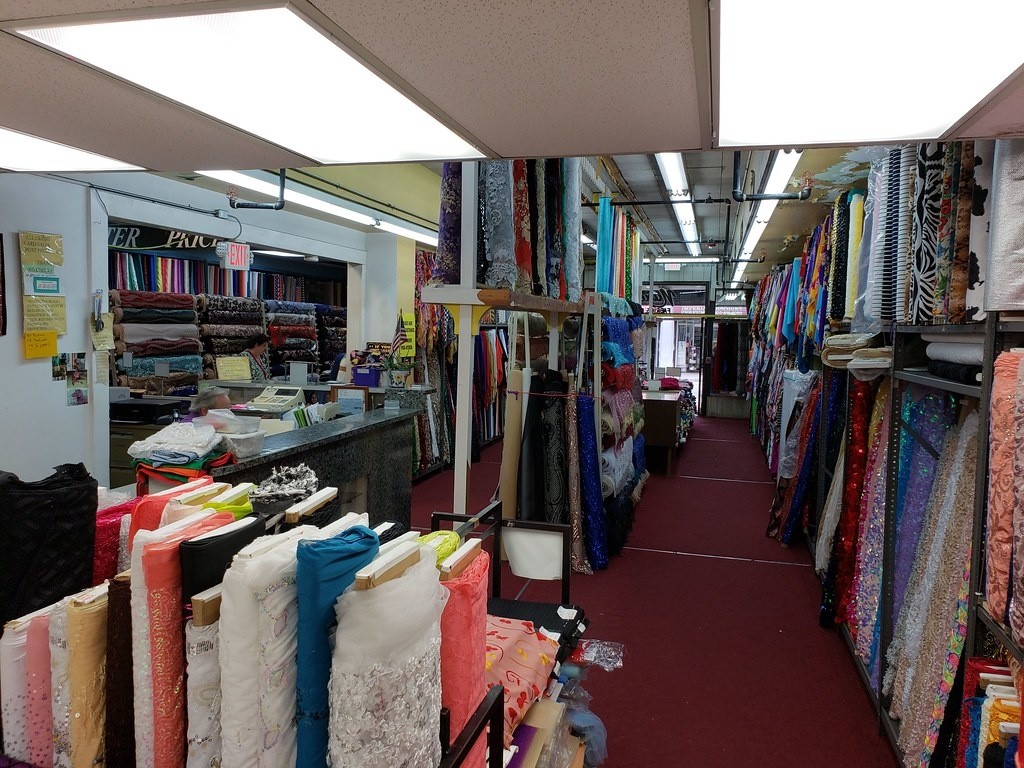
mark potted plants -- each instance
[378,352,421,389]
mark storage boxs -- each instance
[352,366,381,386]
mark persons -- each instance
[239,332,271,384]
[180,386,231,423]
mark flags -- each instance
[389,315,409,355]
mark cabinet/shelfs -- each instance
[842,318,1024,768]
